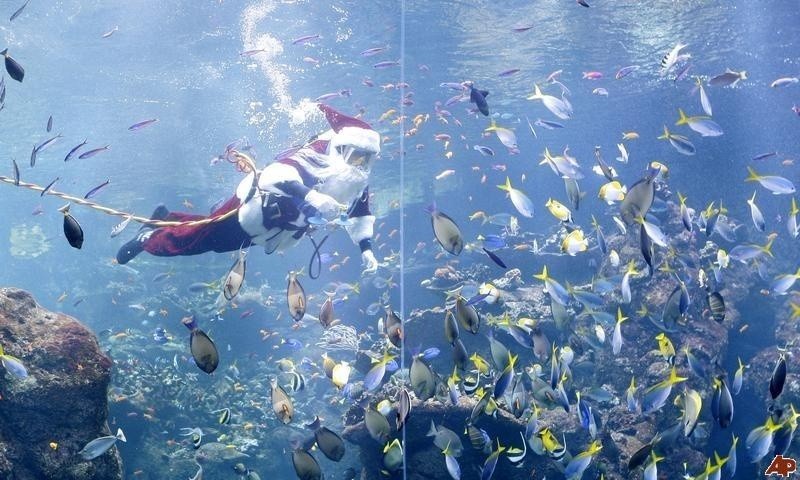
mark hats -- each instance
[316,101,381,153]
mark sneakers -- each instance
[117,205,169,265]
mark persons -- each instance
[116,104,382,275]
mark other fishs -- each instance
[0,0,799,480]
